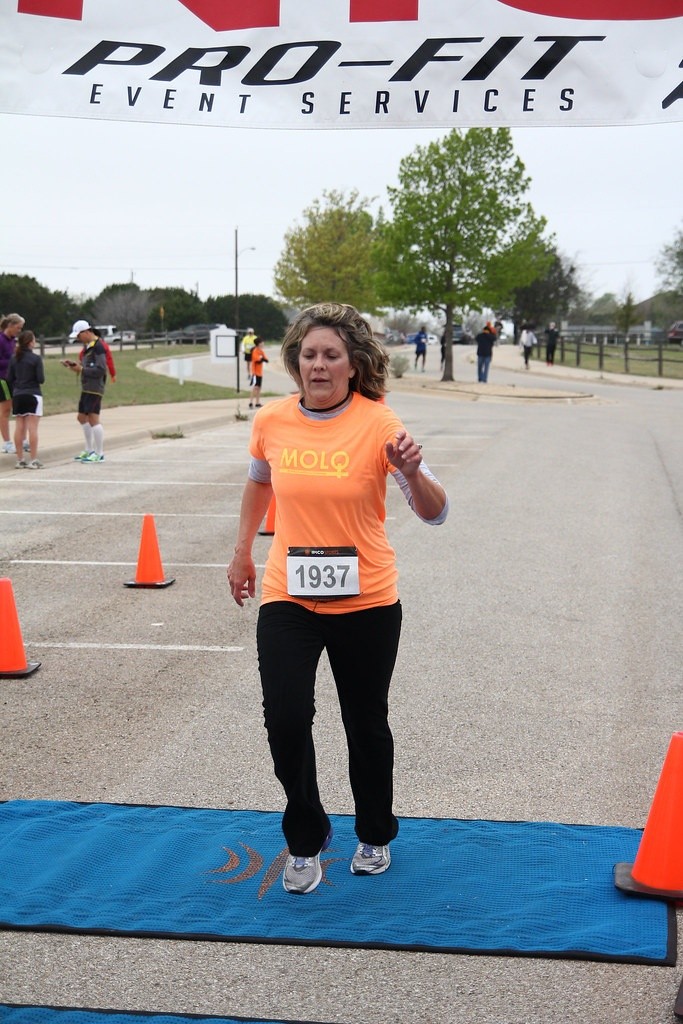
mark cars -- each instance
[405,333,437,345]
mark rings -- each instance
[418,444,422,449]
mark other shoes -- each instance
[255,404,263,407]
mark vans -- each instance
[67,324,117,343]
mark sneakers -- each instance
[29,460,46,468]
[351,842,390,877]
[283,826,332,893]
[74,451,88,461]
[1,441,17,454]
[81,451,104,462]
[15,460,29,468]
[22,439,30,451]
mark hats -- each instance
[69,320,89,338]
[247,328,253,333]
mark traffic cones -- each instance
[614,730,683,901]
[123,512,176,588]
[0,577,43,676]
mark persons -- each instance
[545,322,558,367]
[8,330,45,469]
[225,304,450,894]
[414,326,428,372]
[520,327,537,370]
[60,319,116,464]
[440,331,446,371]
[475,322,496,383]
[242,327,268,408]
[0,313,31,453]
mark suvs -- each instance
[103,330,136,344]
[452,324,466,344]
[667,320,683,346]
[168,323,218,344]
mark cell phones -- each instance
[65,360,74,367]
